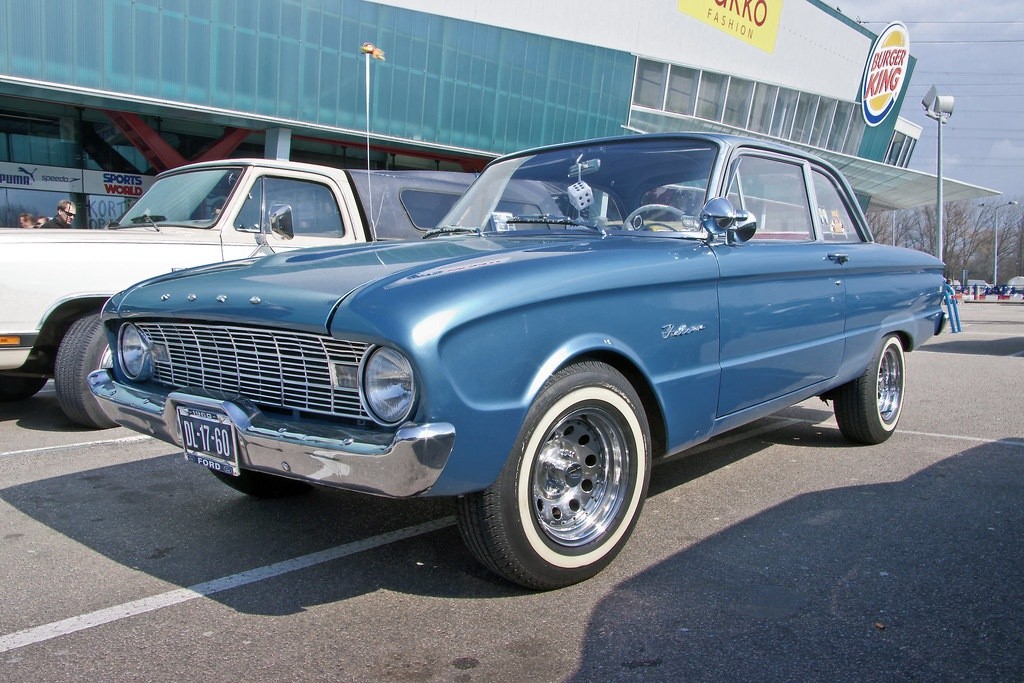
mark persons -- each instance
[211,199,226,215]
[40,199,76,228]
[19,213,48,229]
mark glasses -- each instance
[62,209,76,218]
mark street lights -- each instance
[976,200,1017,287]
[922,85,954,261]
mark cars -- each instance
[87,131,951,591]
[1000,276,1024,295]
[966,278,990,291]
[948,281,962,291]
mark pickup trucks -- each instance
[1,157,564,430]
